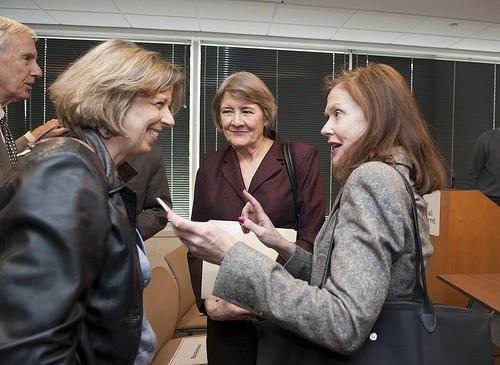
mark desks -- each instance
[437,273,500,315]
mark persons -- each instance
[186,71,326,365]
[122,142,173,241]
[466,128,500,205]
[0,38,187,365]
[165,62,448,353]
[1,16,71,192]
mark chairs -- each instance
[143,244,208,365]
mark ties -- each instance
[0,114,19,169]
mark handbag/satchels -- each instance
[255,168,494,365]
